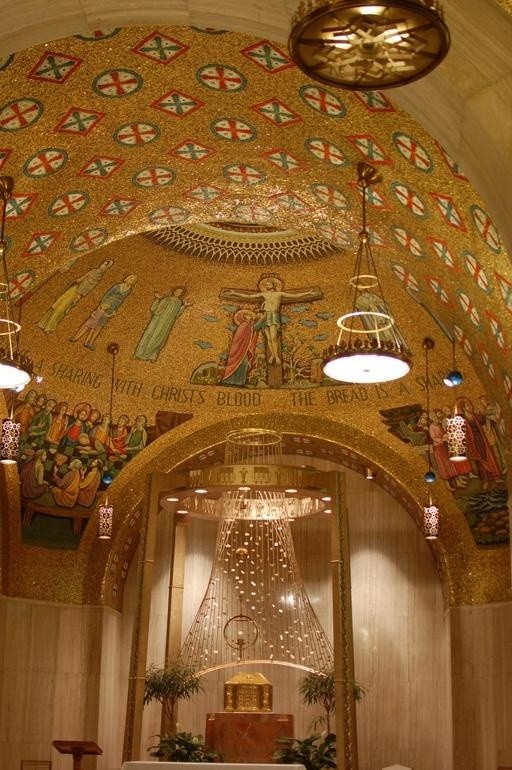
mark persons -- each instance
[129,285,196,363]
[221,304,266,388]
[13,390,150,508]
[68,275,140,351]
[407,396,510,492]
[351,281,406,351]
[33,259,115,333]
[224,276,316,365]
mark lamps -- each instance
[98,344,119,540]
[422,338,467,541]
[1,175,32,466]
[318,159,413,385]
